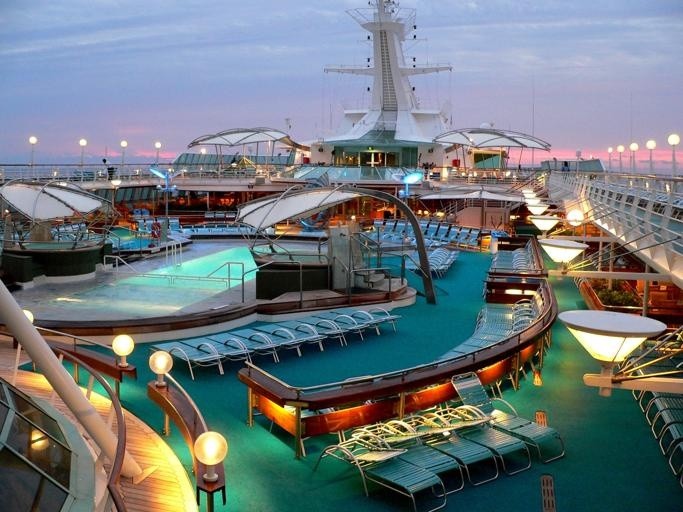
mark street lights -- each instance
[79,139,87,183]
[155,141,161,169]
[120,140,127,180]
[646,140,656,174]
[630,143,638,173]
[391,171,422,235]
[668,134,680,177]
[616,144,625,173]
[607,147,612,173]
[29,136,37,179]
[200,147,206,171]
[148,167,181,218]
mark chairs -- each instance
[488,239,539,274]
[363,217,480,279]
[147,297,569,511]
[51,223,97,243]
[139,217,276,255]
[586,246,630,271]
[620,332,682,489]
[133,208,153,224]
[204,211,237,219]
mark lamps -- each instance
[538,237,589,275]
[523,189,560,215]
[111,334,135,368]
[557,309,668,374]
[528,218,562,235]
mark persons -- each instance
[230,157,237,164]
[560,161,570,179]
[151,218,161,238]
[103,158,114,180]
